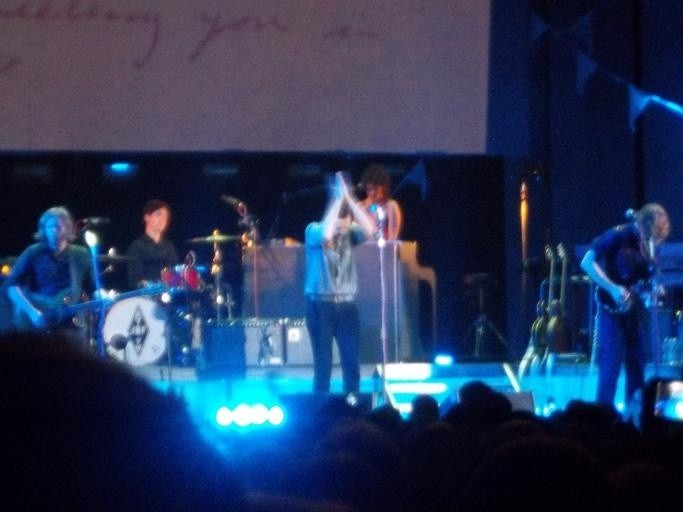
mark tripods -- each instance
[155,241,235,368]
[452,285,520,362]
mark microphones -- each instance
[220,194,242,210]
[84,230,98,256]
[646,234,655,259]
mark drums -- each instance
[162,264,202,296]
[102,297,169,367]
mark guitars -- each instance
[14,284,162,332]
[531,242,573,353]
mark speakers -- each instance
[437,391,533,417]
[285,394,372,426]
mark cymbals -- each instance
[98,255,136,264]
[190,234,239,243]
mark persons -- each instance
[351,167,402,239]
[125,198,179,291]
[3,205,100,357]
[577,201,671,414]
[300,171,378,398]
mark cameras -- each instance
[646,378,683,425]
